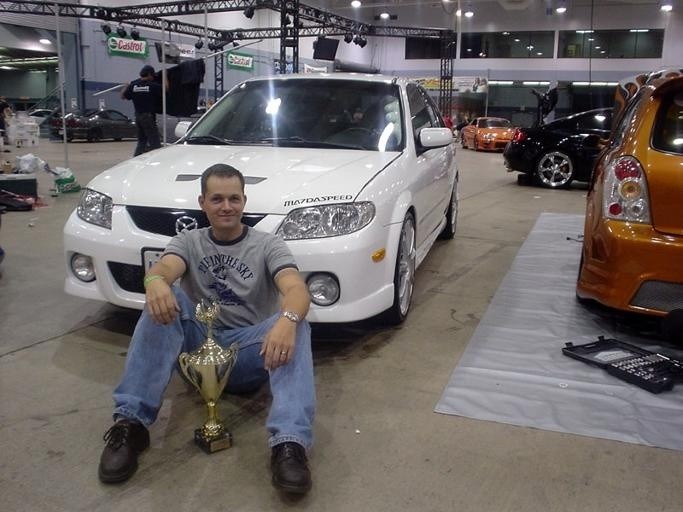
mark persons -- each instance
[97,163,317,496]
[120,65,169,158]
[443,116,454,134]
[0,98,14,153]
[456,109,469,144]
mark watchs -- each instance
[279,309,301,324]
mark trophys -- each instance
[177,296,240,454]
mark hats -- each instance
[143,64,158,77]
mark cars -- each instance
[576,69,682,331]
[63,71,458,328]
[28,109,53,126]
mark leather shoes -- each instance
[271,442,313,495]
[97,418,151,484]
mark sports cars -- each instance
[502,106,614,190]
[66,108,139,142]
[460,117,515,152]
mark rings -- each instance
[280,350,287,357]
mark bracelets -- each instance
[143,273,165,286]
